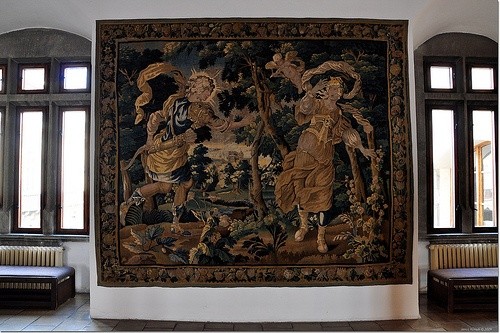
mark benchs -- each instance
[427,268,498,313]
[0,265,76,310]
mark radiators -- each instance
[429,243,499,290]
[0,247,65,290]
